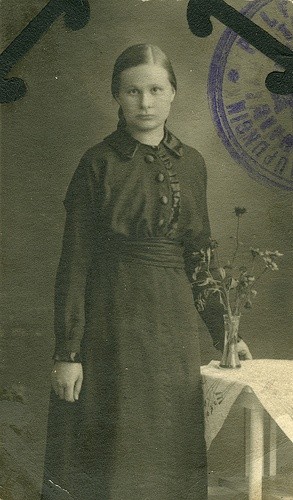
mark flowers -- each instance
[190,205,285,315]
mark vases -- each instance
[220,314,242,368]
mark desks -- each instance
[200,359,293,500]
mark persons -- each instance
[42,44,253,500]
